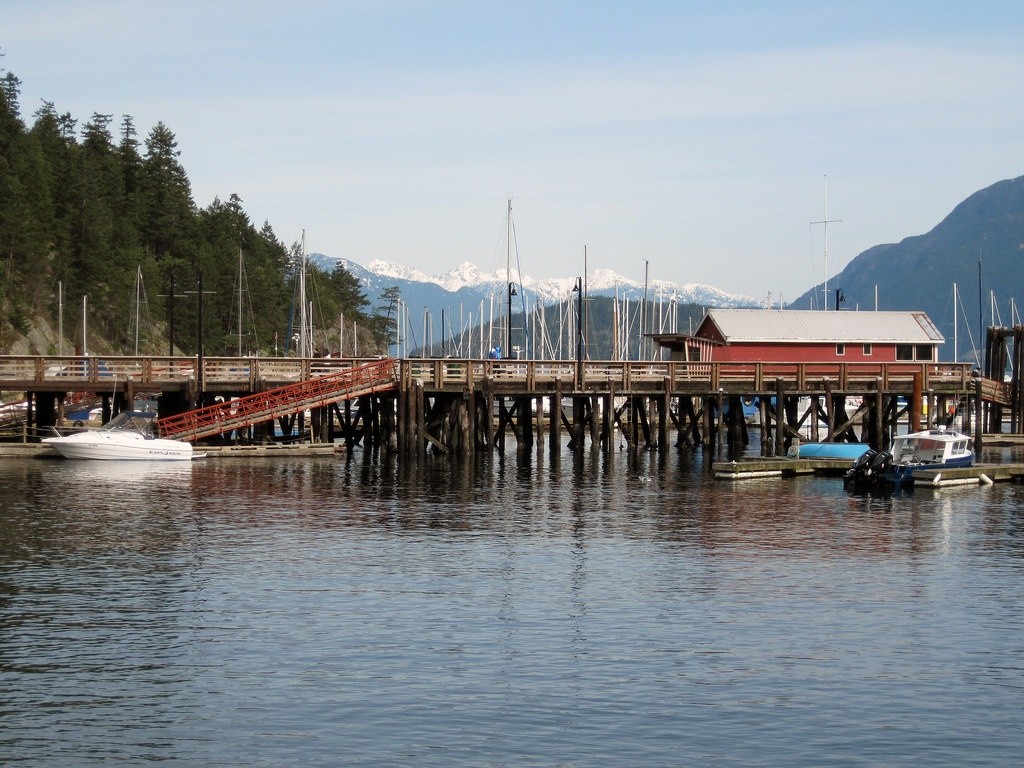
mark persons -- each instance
[489,347,501,379]
[310,348,322,377]
[322,349,331,373]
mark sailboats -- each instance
[1,193,1020,435]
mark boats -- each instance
[39,410,195,462]
[842,425,975,494]
[786,441,870,461]
